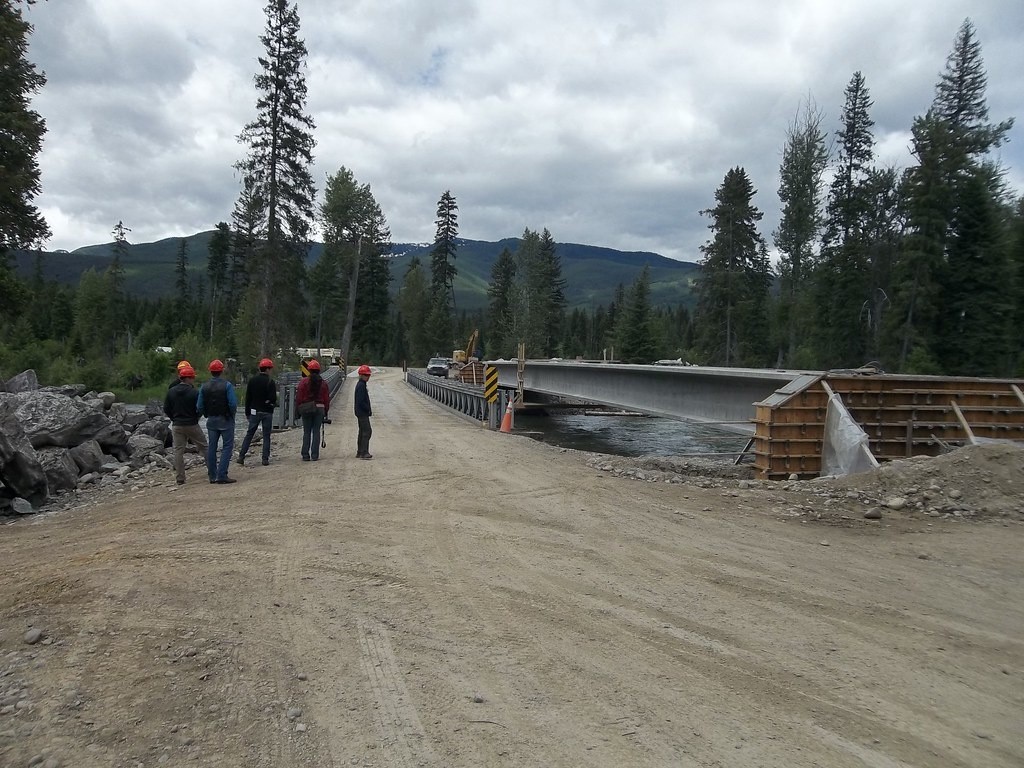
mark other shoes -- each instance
[215,476,237,483]
[303,456,309,460]
[177,480,183,485]
[236,458,244,464]
[210,481,216,483]
[261,459,269,466]
[357,453,372,458]
[312,457,318,461]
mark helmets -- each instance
[308,359,321,369]
[357,365,372,375]
[259,358,273,368]
[179,366,194,377]
[208,359,223,371]
[177,360,191,370]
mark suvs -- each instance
[427,357,450,379]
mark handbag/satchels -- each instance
[298,402,318,419]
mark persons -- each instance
[237,358,277,466]
[168,361,200,440]
[197,360,237,484]
[354,365,373,458]
[293,360,329,461]
[163,367,229,484]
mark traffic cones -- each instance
[497,397,513,434]
